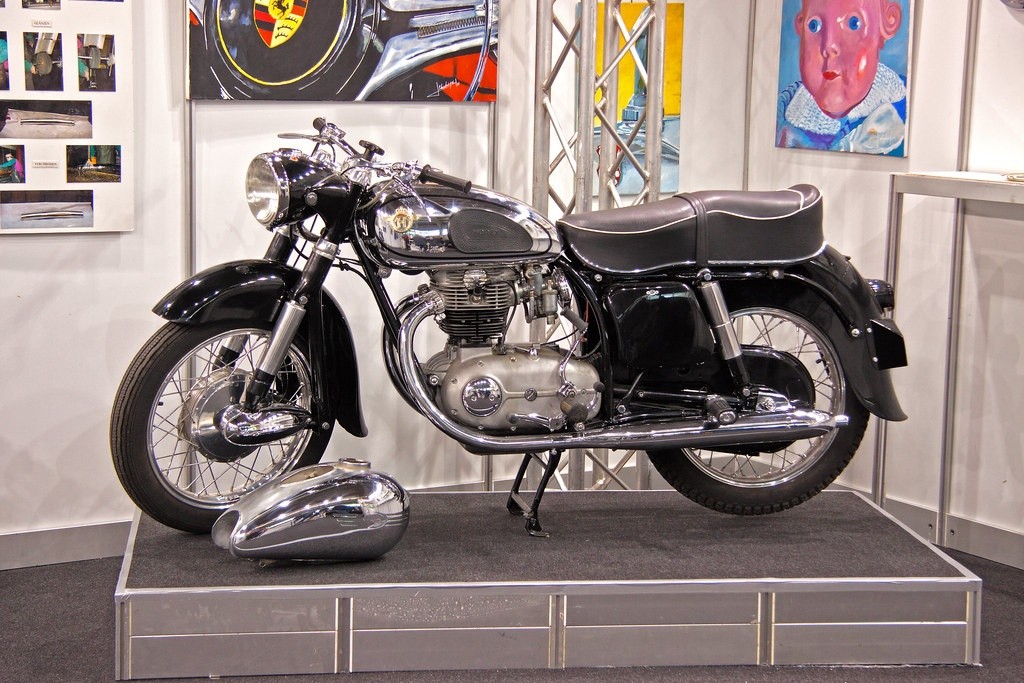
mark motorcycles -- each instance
[109,116,908,537]
